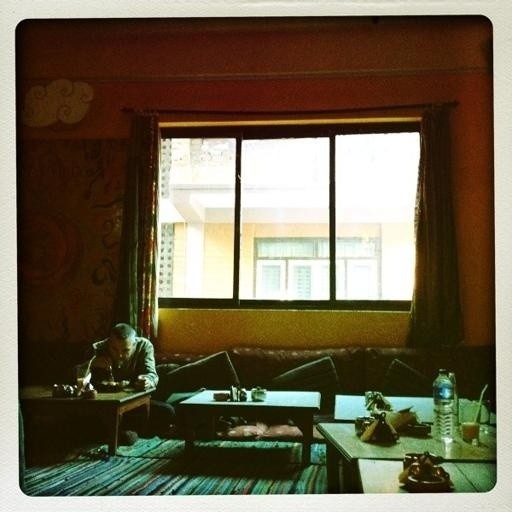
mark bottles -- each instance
[432,368,456,444]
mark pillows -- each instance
[166,353,240,390]
[382,358,434,399]
[267,358,343,416]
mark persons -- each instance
[82,321,178,447]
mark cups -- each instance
[471,399,492,434]
[410,423,430,438]
[457,399,481,449]
[403,452,449,490]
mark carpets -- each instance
[21,435,340,495]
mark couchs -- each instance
[40,346,489,442]
